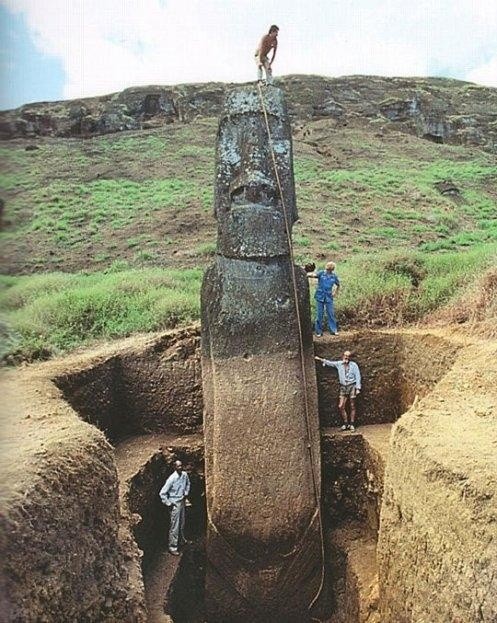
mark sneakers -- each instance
[170,550,180,556]
[330,332,340,336]
[180,540,195,545]
[337,424,356,433]
[315,332,323,338]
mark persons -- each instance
[315,350,362,433]
[254,25,279,87]
[159,460,193,556]
[307,262,340,338]
